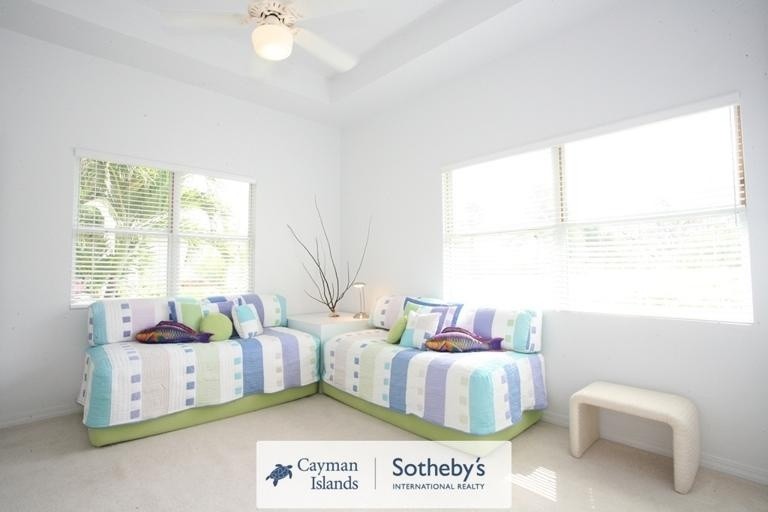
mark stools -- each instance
[353,281,370,319]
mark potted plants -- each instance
[286,193,370,317]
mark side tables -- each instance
[289,311,371,338]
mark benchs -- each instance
[78,293,319,446]
[567,382,698,494]
[324,295,546,456]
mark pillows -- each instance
[230,304,264,338]
[385,315,410,343]
[136,321,213,344]
[400,309,442,348]
[200,314,232,341]
[427,328,504,354]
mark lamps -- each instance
[247,0,302,61]
